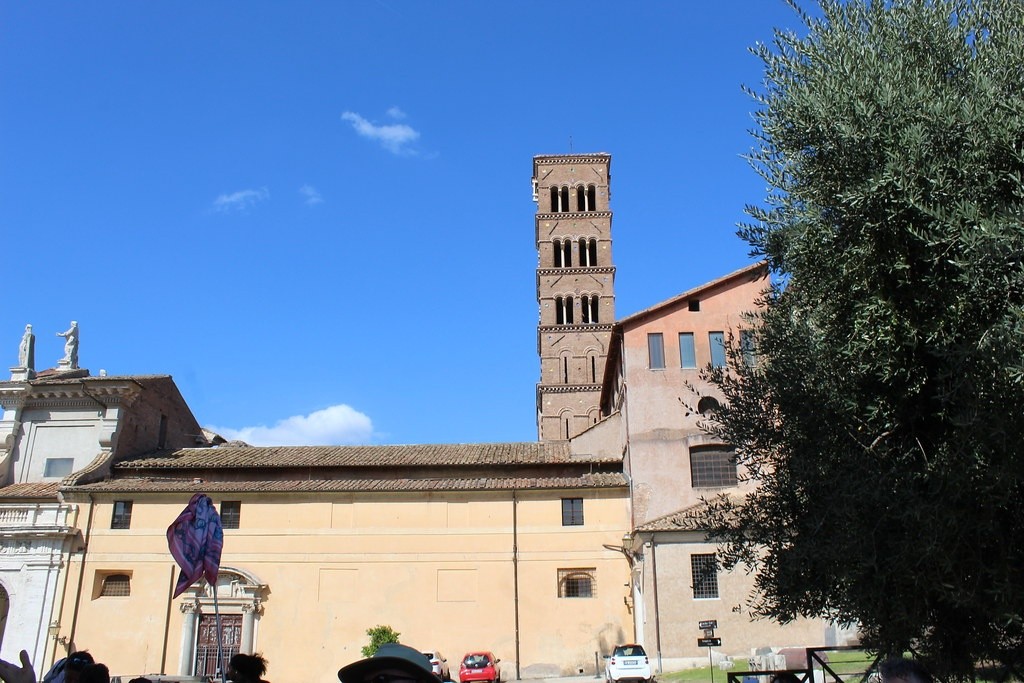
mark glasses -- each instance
[360,674,421,683]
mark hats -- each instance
[338,643,442,683]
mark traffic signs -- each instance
[697,637,722,646]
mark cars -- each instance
[420,649,450,682]
[602,642,652,683]
[458,650,501,683]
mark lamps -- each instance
[622,532,635,567]
[48,620,66,646]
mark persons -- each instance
[129,677,153,683]
[56,321,79,370]
[0,650,110,683]
[18,324,35,370]
[880,657,934,683]
[337,643,442,683]
[224,653,270,683]
[100,369,108,378]
[768,672,804,683]
